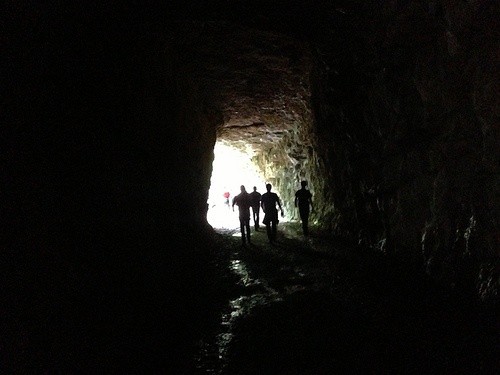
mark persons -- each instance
[249,186,262,231]
[261,183,284,243]
[233,185,253,245]
[294,181,314,237]
